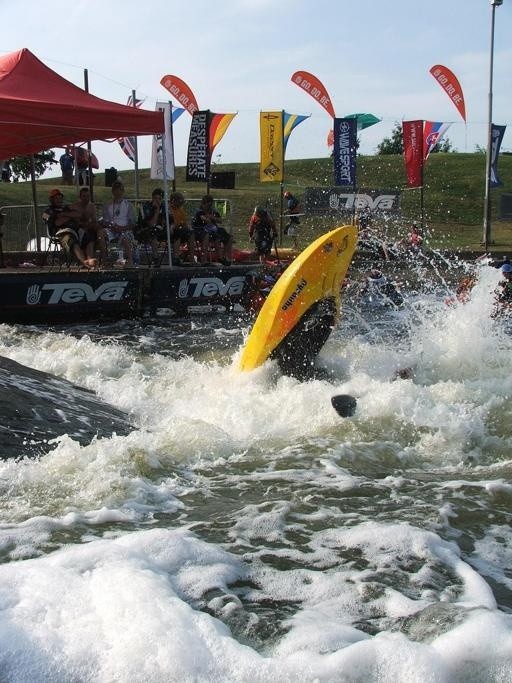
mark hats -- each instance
[49,189,63,198]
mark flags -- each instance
[326,112,383,146]
[422,118,454,160]
[283,112,309,152]
[172,105,186,124]
[208,110,238,158]
[117,94,146,161]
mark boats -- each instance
[239,224,359,372]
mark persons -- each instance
[247,205,279,264]
[68,186,105,266]
[168,189,214,268]
[190,193,235,267]
[133,187,185,268]
[394,220,424,259]
[98,180,138,269]
[281,190,303,250]
[74,160,89,186]
[337,261,512,321]
[59,147,75,186]
[42,188,101,271]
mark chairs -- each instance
[38,219,235,272]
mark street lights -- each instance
[481,1,504,245]
